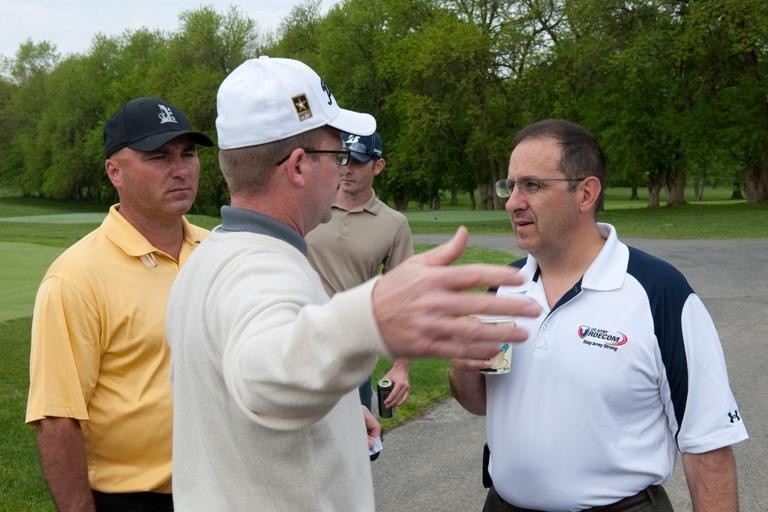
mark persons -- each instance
[448,118,748,512]
[304,131,414,415]
[165,54,541,512]
[24,96,215,512]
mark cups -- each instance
[472,315,513,377]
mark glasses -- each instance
[277,149,351,167]
[496,178,582,198]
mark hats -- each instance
[342,132,382,162]
[215,55,377,149]
[104,96,213,158]
